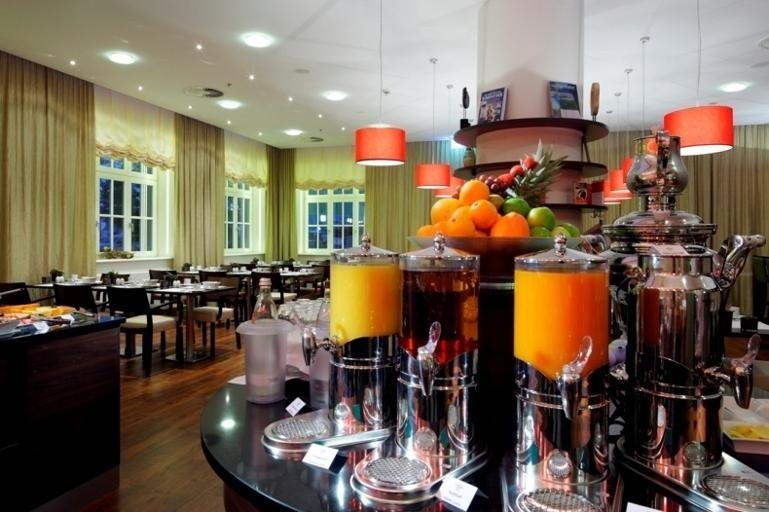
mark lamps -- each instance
[355,1,408,166]
[659,0,741,155]
[433,80,464,197]
[593,32,652,204]
[415,56,452,191]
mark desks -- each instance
[201,368,767,511]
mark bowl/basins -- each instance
[172,278,221,292]
[408,237,587,276]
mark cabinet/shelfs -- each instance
[0,309,127,512]
[454,110,611,211]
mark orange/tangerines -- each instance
[490,191,580,238]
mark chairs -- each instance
[2,282,31,305]
[27,260,330,376]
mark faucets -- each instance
[416,321,441,398]
[301,326,338,368]
[555,336,595,420]
[703,333,762,409]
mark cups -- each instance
[114,276,158,289]
[236,318,290,405]
[279,297,330,324]
[178,257,324,274]
[54,272,96,285]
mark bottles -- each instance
[308,292,329,411]
[251,278,276,320]
[462,146,475,168]
